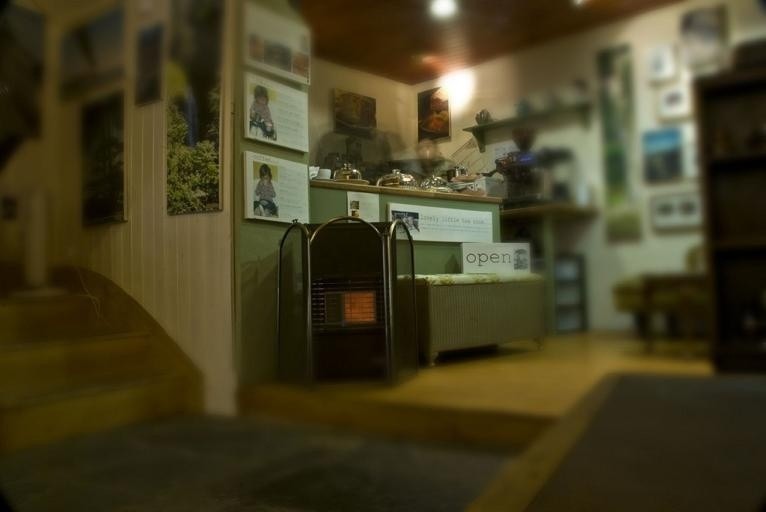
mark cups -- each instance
[317,169,331,178]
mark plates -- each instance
[419,117,440,134]
[335,89,375,131]
[315,178,332,181]
[333,179,370,185]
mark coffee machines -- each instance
[494,153,554,205]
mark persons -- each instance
[249,83,274,138]
[253,161,278,217]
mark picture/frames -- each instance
[642,6,735,231]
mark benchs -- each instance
[400,274,554,365]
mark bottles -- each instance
[447,166,465,180]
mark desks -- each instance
[498,199,600,336]
[309,179,504,275]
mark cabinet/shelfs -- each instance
[694,66,765,381]
